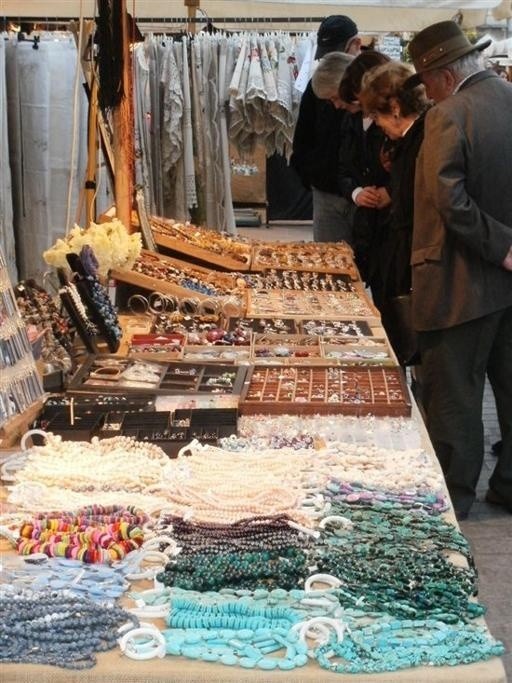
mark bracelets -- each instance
[129,288,241,318]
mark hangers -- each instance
[131,15,322,42]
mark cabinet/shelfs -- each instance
[0,242,506,682]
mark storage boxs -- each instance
[252,333,321,359]
[45,395,155,409]
[47,406,242,455]
[228,316,298,336]
[240,364,412,417]
[152,314,224,334]
[177,331,253,364]
[142,212,253,272]
[321,336,393,361]
[244,288,380,322]
[245,268,357,293]
[68,353,248,400]
[115,249,245,314]
[128,333,186,360]
[300,319,374,337]
[253,238,355,276]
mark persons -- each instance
[289,13,363,242]
[312,52,363,115]
[400,19,512,524]
[358,60,431,354]
[338,50,400,274]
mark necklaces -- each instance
[61,282,97,340]
[0,411,505,674]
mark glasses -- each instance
[317,38,355,62]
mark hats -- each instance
[400,20,492,89]
[313,14,360,61]
